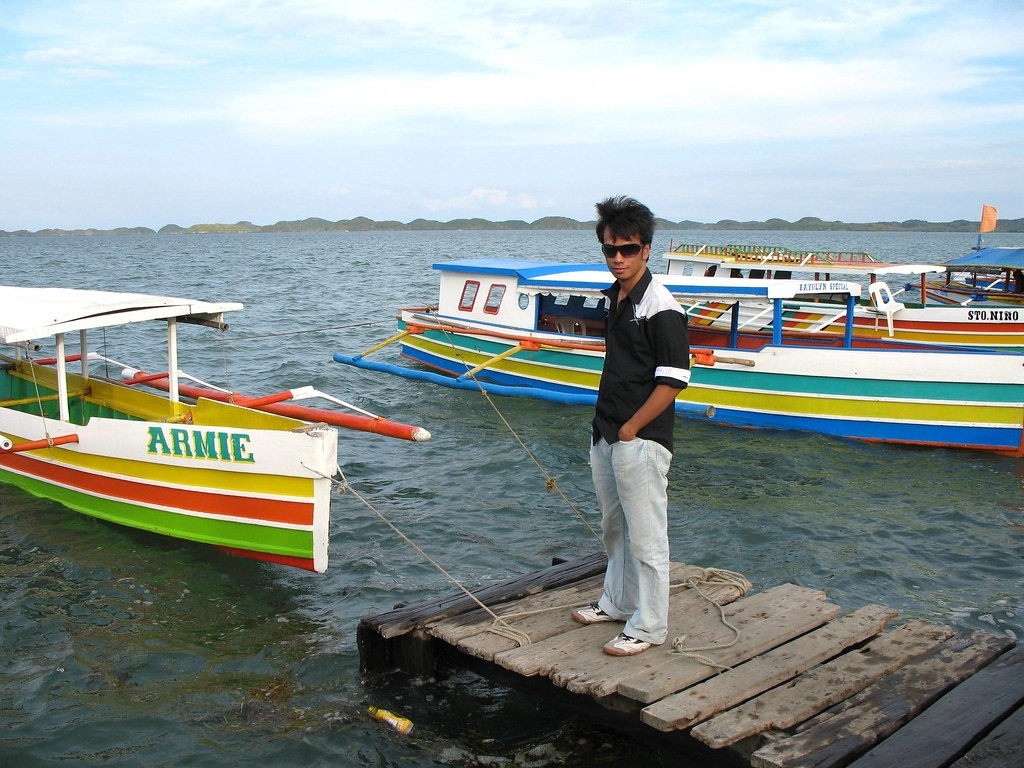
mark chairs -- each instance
[554,317,588,336]
[868,281,906,338]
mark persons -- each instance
[1013,270,1024,294]
[572,197,691,657]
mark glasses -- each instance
[601,243,645,258]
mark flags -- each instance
[980,206,997,233]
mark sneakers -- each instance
[603,632,652,656]
[572,601,616,624]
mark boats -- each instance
[331,232,1024,460]
[1,284,434,575]
